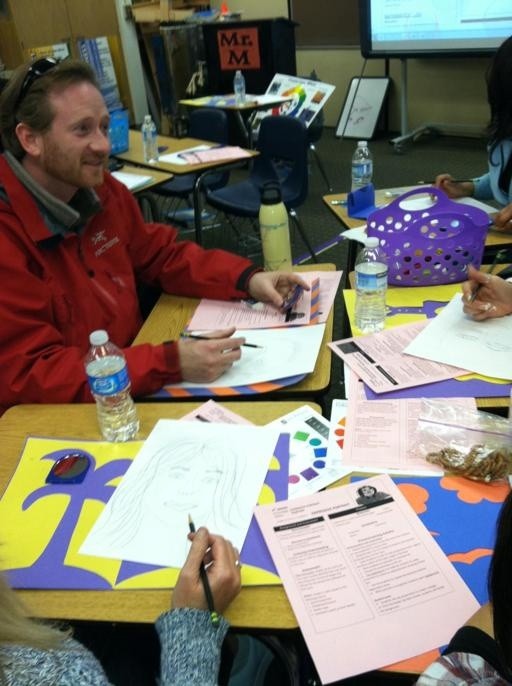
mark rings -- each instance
[508,218,512,224]
[233,559,242,569]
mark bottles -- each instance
[354,237,389,332]
[141,115,159,163]
[83,330,141,442]
[350,141,375,194]
[234,70,246,102]
[257,184,293,273]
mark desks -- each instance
[114,128,261,246]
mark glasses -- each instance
[20,56,60,96]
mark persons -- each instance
[428,34,512,239]
[1,525,295,686]
[2,57,312,415]
[456,259,512,323]
[412,485,511,686]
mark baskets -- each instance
[109,106,129,157]
[367,187,488,287]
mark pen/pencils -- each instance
[417,180,480,184]
[331,200,349,205]
[469,253,502,306]
[384,191,410,198]
[188,514,219,628]
[180,333,264,349]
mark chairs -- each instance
[151,76,334,264]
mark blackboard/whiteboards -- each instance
[335,76,390,140]
[287,0,365,50]
